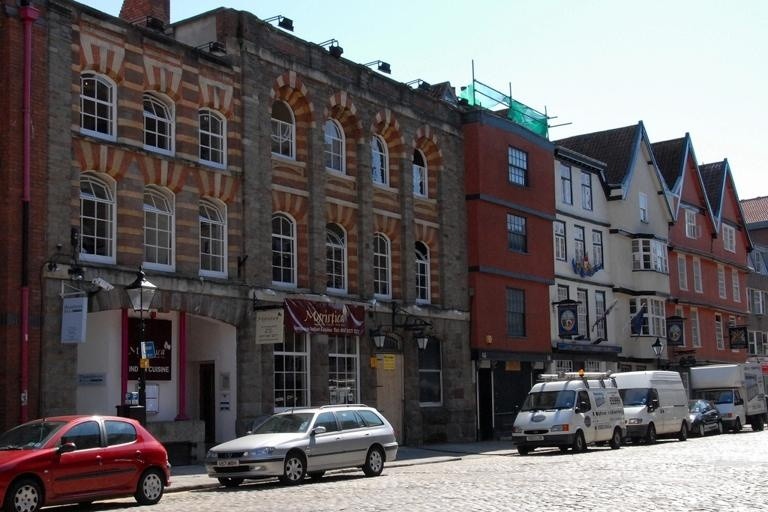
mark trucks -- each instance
[511,362,768,454]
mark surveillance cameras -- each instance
[91,277,115,292]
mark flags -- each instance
[630,306,645,343]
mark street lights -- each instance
[651,337,666,371]
[125,265,158,425]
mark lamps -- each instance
[411,328,431,355]
[370,328,386,353]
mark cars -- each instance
[0,414,174,511]
[204,401,397,489]
